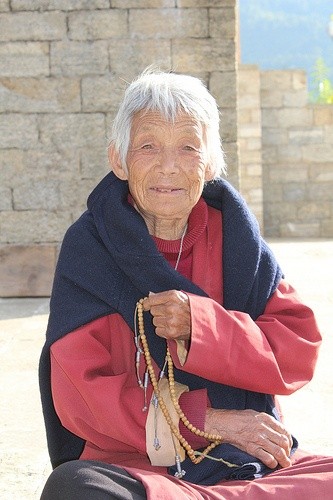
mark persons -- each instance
[38,72,333,500]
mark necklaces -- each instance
[143,219,191,272]
[131,302,188,479]
[135,297,224,464]
